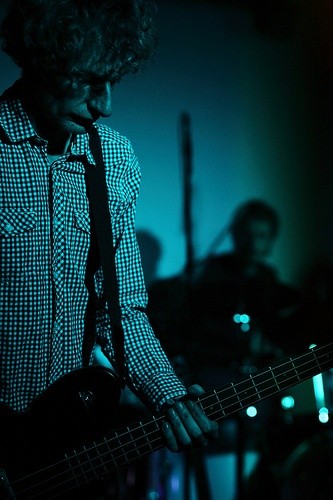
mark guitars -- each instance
[0,343,333,500]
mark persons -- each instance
[0,0,220,500]
[186,201,333,372]
